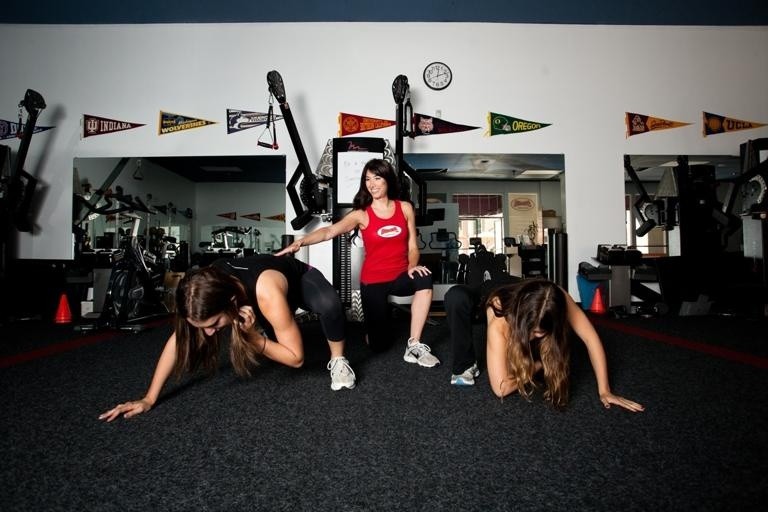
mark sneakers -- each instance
[404,340,441,368]
[330,357,356,392]
[451,364,480,386]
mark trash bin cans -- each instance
[577,245,631,319]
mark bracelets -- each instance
[256,334,269,356]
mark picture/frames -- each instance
[624,155,741,259]
[393,154,566,284]
[71,155,286,272]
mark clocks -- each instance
[423,62,452,91]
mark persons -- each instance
[273,158,441,369]
[98,253,355,424]
[443,274,647,415]
